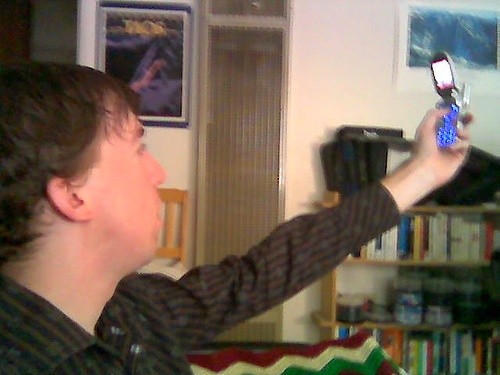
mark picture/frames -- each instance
[95,2,193,127]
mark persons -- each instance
[0,58,477,375]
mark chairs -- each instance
[151,188,189,277]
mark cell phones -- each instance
[429,52,471,150]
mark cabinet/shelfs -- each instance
[312,192,499,375]
